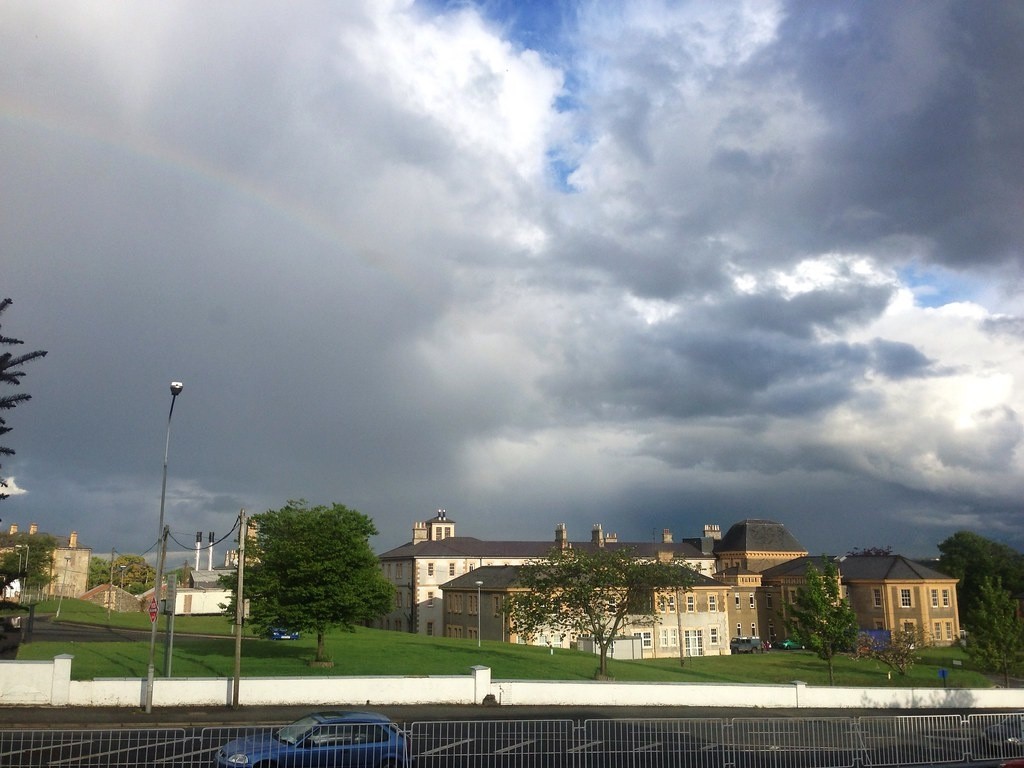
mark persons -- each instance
[765,640,771,653]
[759,641,765,653]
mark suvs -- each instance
[730,636,761,654]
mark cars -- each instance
[212,711,411,768]
[266,626,300,641]
[978,710,1024,755]
[778,637,806,650]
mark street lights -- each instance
[16,545,29,603]
[119,566,126,612]
[145,380,183,713]
[55,556,71,617]
[475,581,483,648]
